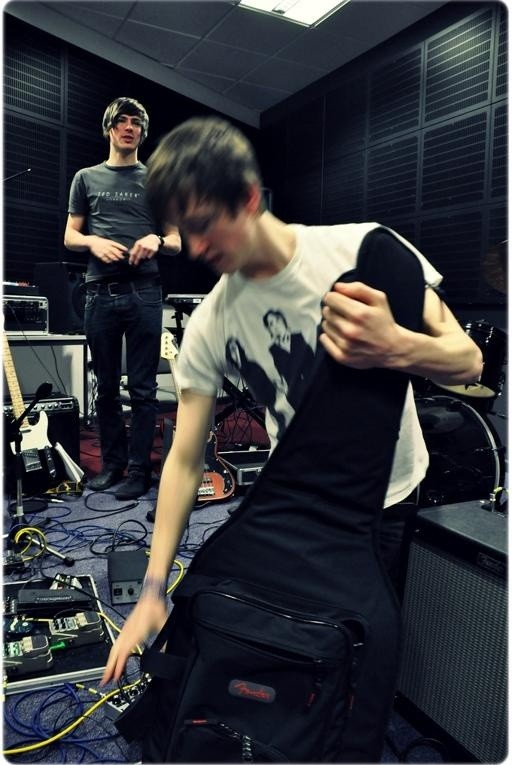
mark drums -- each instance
[425,319,508,400]
[416,394,505,509]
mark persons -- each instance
[261,309,315,413]
[98,116,484,690]
[63,97,183,501]
[225,335,287,442]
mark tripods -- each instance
[2,381,75,567]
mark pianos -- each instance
[165,294,207,304]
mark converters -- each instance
[108,550,148,605]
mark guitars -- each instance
[4,330,66,490]
[160,327,235,501]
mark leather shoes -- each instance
[114,472,153,500]
[90,469,123,490]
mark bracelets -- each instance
[157,234,165,247]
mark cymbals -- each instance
[483,240,507,294]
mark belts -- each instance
[87,277,156,297]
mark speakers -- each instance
[391,496,508,764]
[33,261,88,335]
